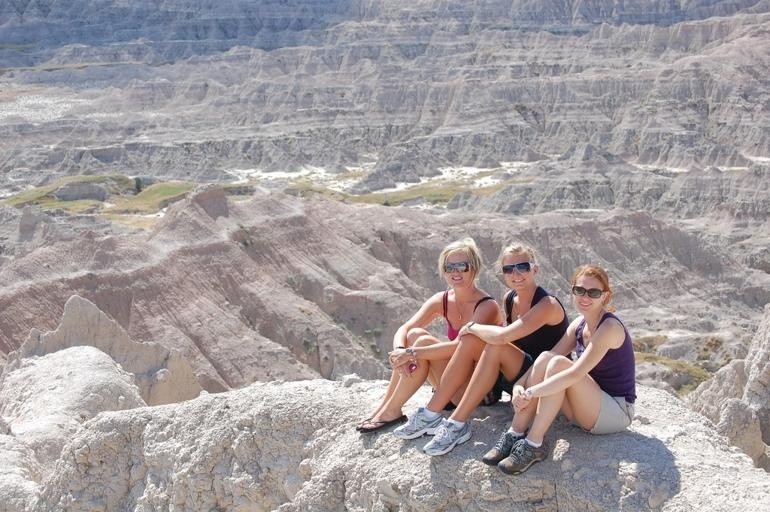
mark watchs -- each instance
[405,345,417,362]
[465,320,476,334]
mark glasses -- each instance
[444,261,473,274]
[502,262,534,274]
[571,285,606,298]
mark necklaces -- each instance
[454,290,473,321]
[513,293,531,319]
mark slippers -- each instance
[354,416,373,431]
[361,415,408,434]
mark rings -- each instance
[391,358,394,363]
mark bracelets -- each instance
[526,387,533,398]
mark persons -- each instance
[481,263,637,474]
[355,236,507,434]
[393,243,570,455]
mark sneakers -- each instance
[498,438,547,475]
[422,418,473,457]
[482,429,528,466]
[392,406,444,441]
